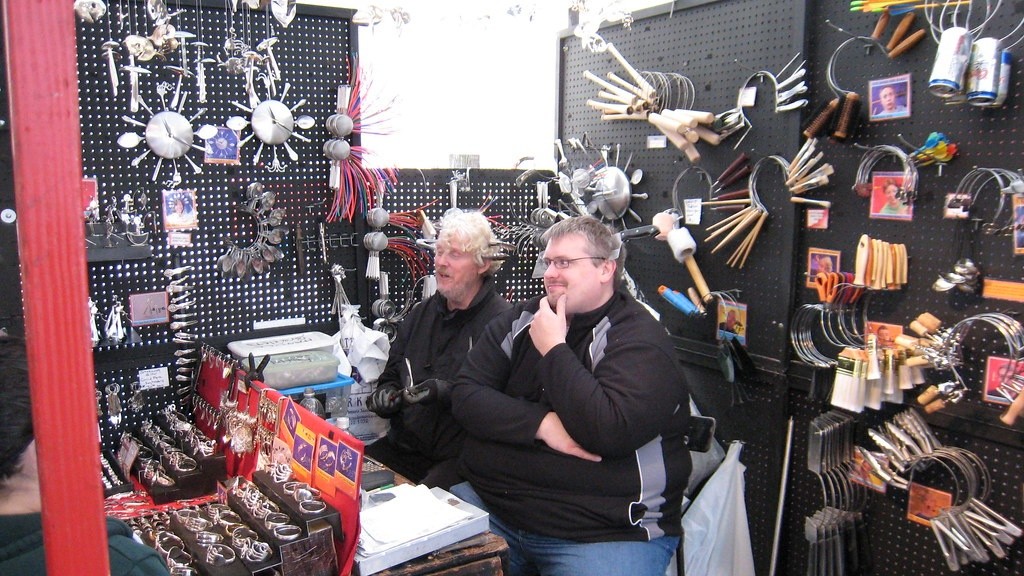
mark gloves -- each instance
[366,390,406,419]
[403,379,452,406]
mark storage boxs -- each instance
[354,486,489,576]
[227,331,355,416]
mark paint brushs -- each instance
[702,137,835,269]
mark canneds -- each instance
[928,27,1014,107]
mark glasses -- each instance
[543,256,609,270]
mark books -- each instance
[352,486,490,576]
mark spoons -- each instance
[588,201,597,214]
[294,115,315,129]
[193,124,217,139]
[630,169,643,184]
[226,116,250,130]
[117,132,145,148]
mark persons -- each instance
[915,494,938,521]
[449,216,691,576]
[364,209,514,486]
[877,85,907,115]
[876,325,895,346]
[813,255,833,273]
[0,327,171,576]
[851,459,873,483]
[166,197,194,224]
[880,178,910,215]
[987,364,1019,401]
[719,310,738,334]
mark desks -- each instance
[359,464,510,576]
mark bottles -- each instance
[337,417,355,437]
[299,388,326,420]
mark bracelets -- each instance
[117,404,326,576]
